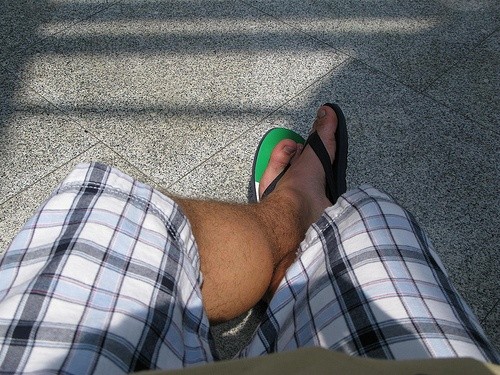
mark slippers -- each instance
[248,129,306,208]
[300,102,347,203]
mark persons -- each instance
[0,103,500,375]
